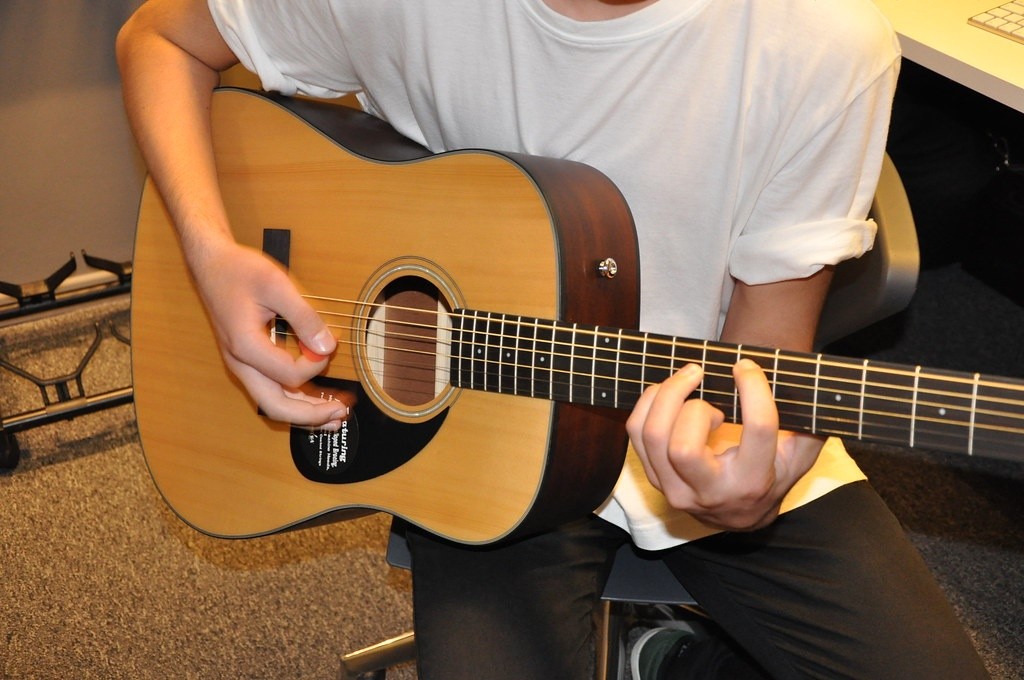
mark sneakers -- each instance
[629,626,701,680]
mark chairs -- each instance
[342,151,920,680]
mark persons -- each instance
[113,0,993,680]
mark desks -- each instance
[870,0,1024,113]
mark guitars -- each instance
[126,88,1024,546]
